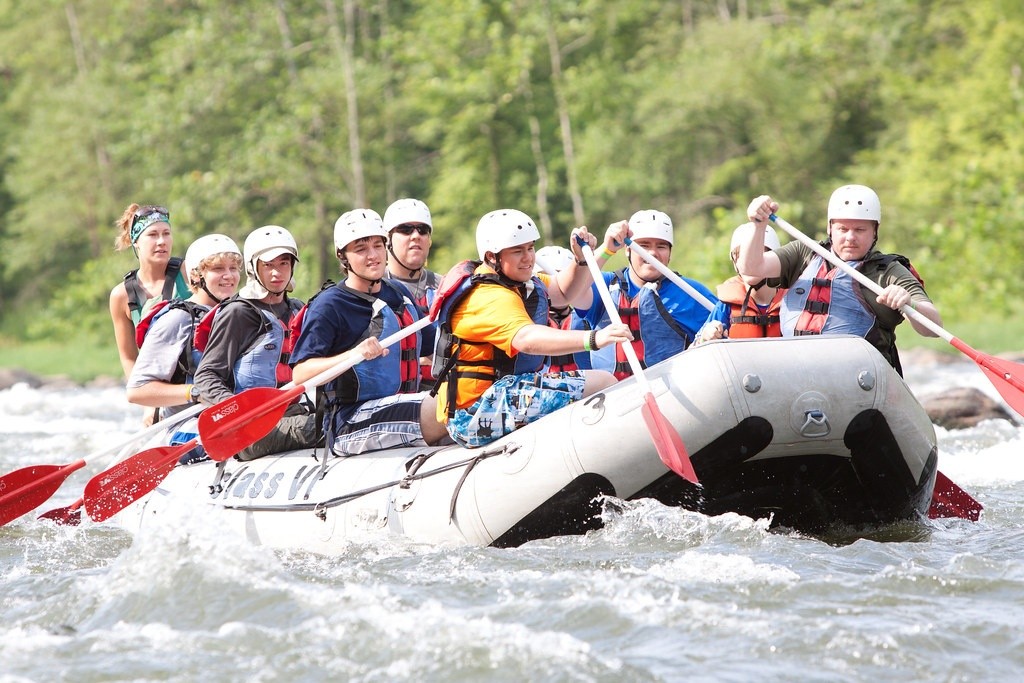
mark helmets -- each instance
[729,222,781,261]
[536,246,576,274]
[334,209,391,261]
[826,185,882,235]
[476,209,541,261]
[625,210,674,257]
[243,225,300,277]
[185,234,243,285]
[383,198,434,233]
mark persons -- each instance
[109,203,195,426]
[195,224,327,462]
[287,208,451,457]
[431,209,635,448]
[571,209,719,380]
[531,246,575,372]
[689,221,801,351]
[384,198,444,390]
[738,183,943,378]
[126,234,242,465]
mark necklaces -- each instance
[389,269,422,300]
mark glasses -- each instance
[130,206,169,233]
[391,223,432,236]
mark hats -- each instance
[258,248,299,263]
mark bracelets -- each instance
[701,339,704,343]
[602,242,617,255]
[575,257,588,266]
[912,301,915,309]
[584,330,600,351]
[186,384,192,403]
[599,248,611,259]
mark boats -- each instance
[115,332,943,573]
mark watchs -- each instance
[191,387,200,403]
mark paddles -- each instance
[615,236,713,311]
[197,311,441,462]
[83,436,202,523]
[37,496,84,526]
[575,234,700,488]
[768,213,1024,416]
[0,403,208,527]
[927,469,984,522]
